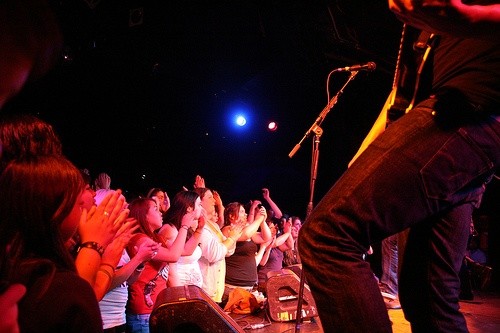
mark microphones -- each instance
[338,62,376,72]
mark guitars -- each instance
[346,1,449,167]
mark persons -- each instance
[458,215,492,300]
[377,234,401,309]
[0,122,301,332]
[298,0,499,333]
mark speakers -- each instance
[266,269,319,322]
[149,285,246,333]
[282,263,308,284]
